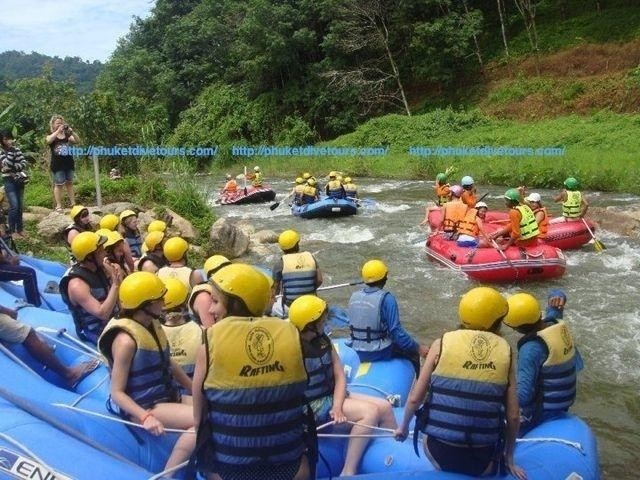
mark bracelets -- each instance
[140,412,153,425]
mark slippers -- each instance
[72,358,100,389]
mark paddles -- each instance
[274,281,364,298]
[491,239,517,271]
[581,216,607,252]
[345,196,374,206]
[270,189,295,211]
[244,166,248,196]
[411,232,437,245]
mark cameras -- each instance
[8,140,17,145]
[61,125,70,132]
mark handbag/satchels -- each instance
[13,171,29,185]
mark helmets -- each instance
[70,205,541,332]
[437,173,578,210]
[296,171,352,186]
[225,173,232,180]
[254,165,261,172]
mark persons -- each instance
[288,294,399,476]
[0,221,42,307]
[502,288,576,439]
[98,271,198,480]
[0,128,30,237]
[221,175,239,195]
[45,115,80,212]
[0,304,100,388]
[60,205,231,395]
[394,287,527,480]
[246,166,262,187]
[293,171,357,206]
[420,173,588,250]
[192,262,312,480]
[271,230,323,295]
[348,259,430,380]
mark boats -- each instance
[425,211,595,282]
[290,195,358,218]
[217,185,276,205]
